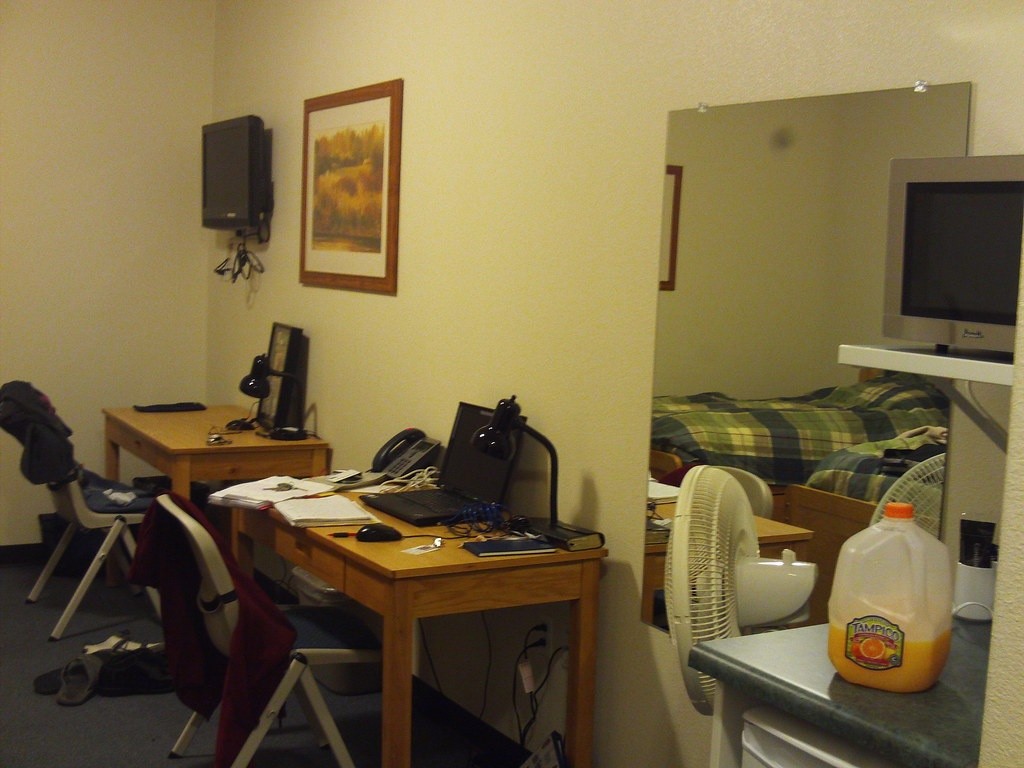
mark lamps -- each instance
[239,354,307,441]
[470,396,606,552]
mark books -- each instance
[464,539,558,556]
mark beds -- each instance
[648,361,949,629]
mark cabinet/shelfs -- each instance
[687,344,1014,768]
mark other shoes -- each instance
[147,648,179,695]
[96,647,147,696]
[82,633,146,656]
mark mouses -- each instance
[356,523,402,542]
[207,434,221,445]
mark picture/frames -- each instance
[257,321,304,431]
[658,165,683,292]
[298,78,403,297]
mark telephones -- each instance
[372,427,441,480]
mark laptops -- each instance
[359,400,525,528]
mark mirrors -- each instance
[641,82,971,637]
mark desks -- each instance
[102,406,330,587]
[642,477,815,624]
[232,471,609,768]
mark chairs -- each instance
[0,381,164,643]
[713,465,774,520]
[149,492,387,768]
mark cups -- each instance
[957,561,996,620]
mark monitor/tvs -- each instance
[201,115,270,228]
[256,321,308,440]
[883,154,1024,359]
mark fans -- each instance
[664,466,818,717]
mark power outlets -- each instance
[535,615,553,658]
[550,640,570,664]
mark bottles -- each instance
[828,502,954,693]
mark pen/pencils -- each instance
[328,533,356,537]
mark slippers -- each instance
[56,655,102,705]
[34,667,63,694]
